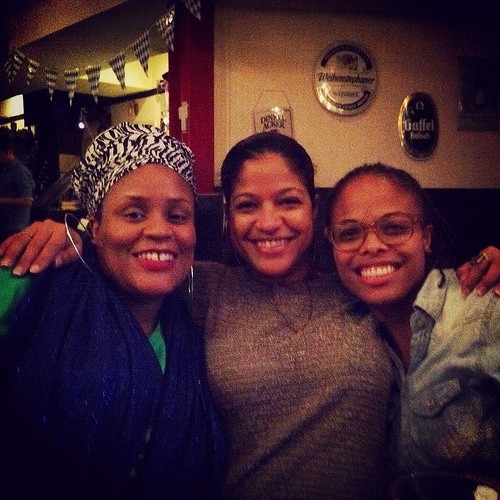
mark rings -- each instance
[476,251,492,263]
[20,233,33,237]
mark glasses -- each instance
[323,217,424,253]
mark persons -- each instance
[0,133,500,500]
[0,131,34,238]
[325,162,500,500]
[0,124,233,500]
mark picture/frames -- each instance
[456,56,500,132]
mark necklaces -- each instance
[277,280,313,331]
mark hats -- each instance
[71,122,197,220]
